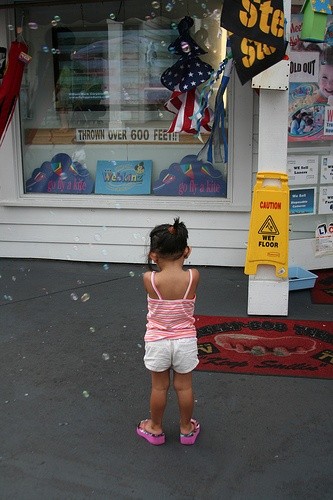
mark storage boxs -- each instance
[289,265,318,290]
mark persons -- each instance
[136,217,200,445]
[314,64,333,107]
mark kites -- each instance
[163,16,212,142]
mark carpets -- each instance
[192,314,333,380]
[310,272,333,304]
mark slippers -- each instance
[137,419,164,445]
[178,415,200,445]
